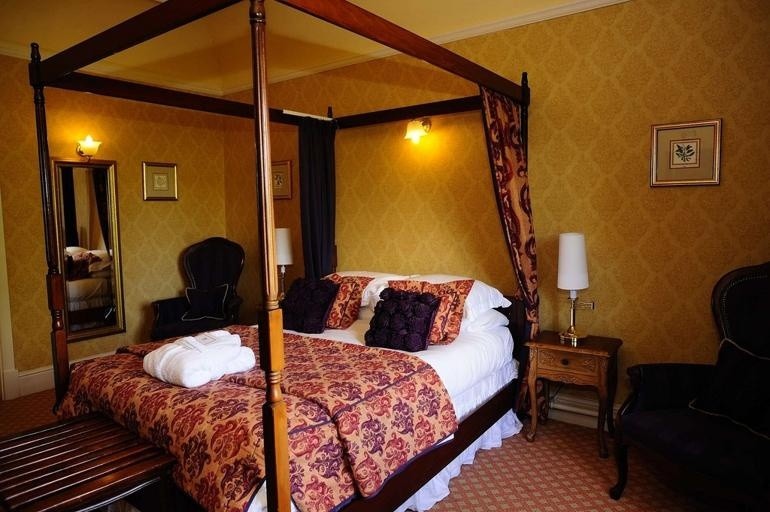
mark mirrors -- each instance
[51,155,124,345]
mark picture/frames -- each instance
[140,160,180,201]
[648,118,722,190]
[270,158,294,201]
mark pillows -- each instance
[63,246,113,281]
[687,338,769,441]
[181,281,230,322]
[282,270,511,354]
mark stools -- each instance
[0,410,176,512]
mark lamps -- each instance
[77,131,103,161]
[557,232,590,347]
[273,226,295,297]
[403,117,433,142]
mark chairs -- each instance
[610,260,769,512]
[150,236,245,339]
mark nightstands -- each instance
[525,330,623,458]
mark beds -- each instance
[28,0,539,512]
[65,278,112,311]
